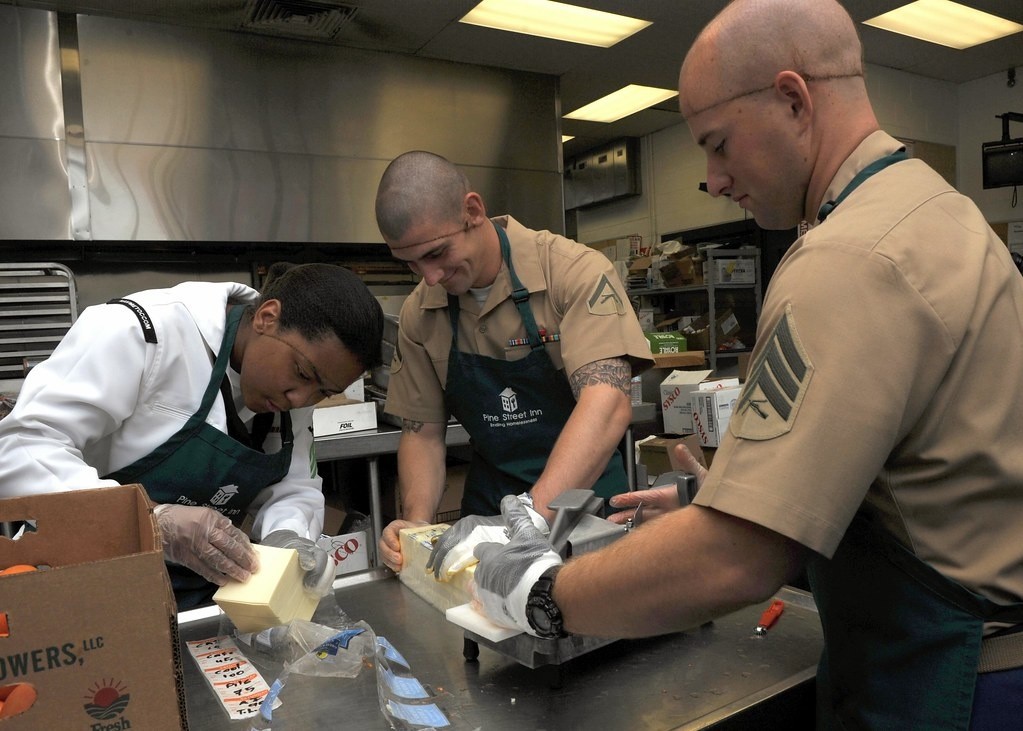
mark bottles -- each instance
[630,374,642,406]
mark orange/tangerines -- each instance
[0,565,40,722]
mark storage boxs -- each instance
[656,316,701,331]
[319,507,375,578]
[638,307,665,324]
[653,237,694,261]
[644,330,687,354]
[312,401,378,438]
[322,496,348,536]
[630,257,652,276]
[0,482,192,731]
[679,308,740,351]
[379,453,471,523]
[344,379,365,402]
[660,369,739,435]
[703,259,755,285]
[638,432,708,476]
[658,260,701,287]
[690,383,744,447]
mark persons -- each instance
[0,262,386,602]
[378,151,657,582]
[473,0,1023,731]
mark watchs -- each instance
[525,565,574,640]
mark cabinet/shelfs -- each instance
[626,247,763,378]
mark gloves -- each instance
[257,530,337,599]
[472,494,563,640]
[423,493,551,584]
